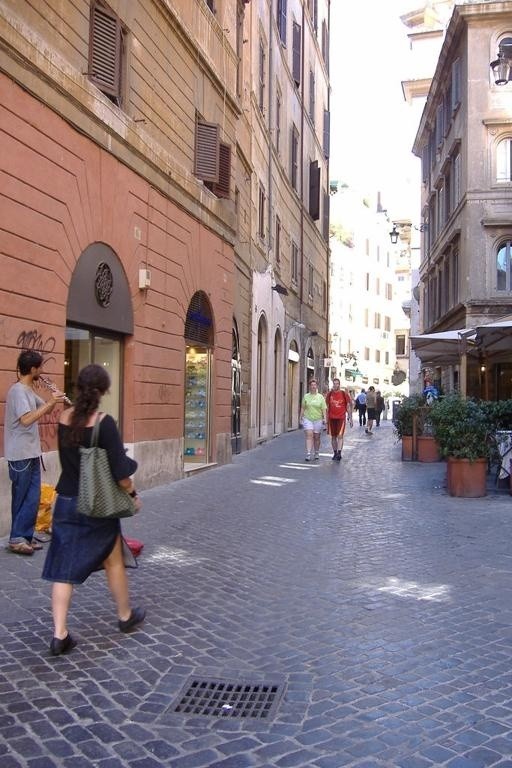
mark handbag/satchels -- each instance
[76,446,135,519]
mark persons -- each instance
[354,386,385,433]
[4,349,66,553]
[300,380,327,461]
[325,378,353,460]
[41,364,146,655]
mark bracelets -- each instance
[129,490,138,498]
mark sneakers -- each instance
[8,542,43,554]
[332,454,341,461]
[305,453,311,460]
[314,451,319,459]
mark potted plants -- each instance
[394,393,512,498]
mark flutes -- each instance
[38,375,73,405]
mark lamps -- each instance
[390,223,429,243]
[490,44,512,85]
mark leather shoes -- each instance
[119,607,145,631]
[50,633,77,656]
